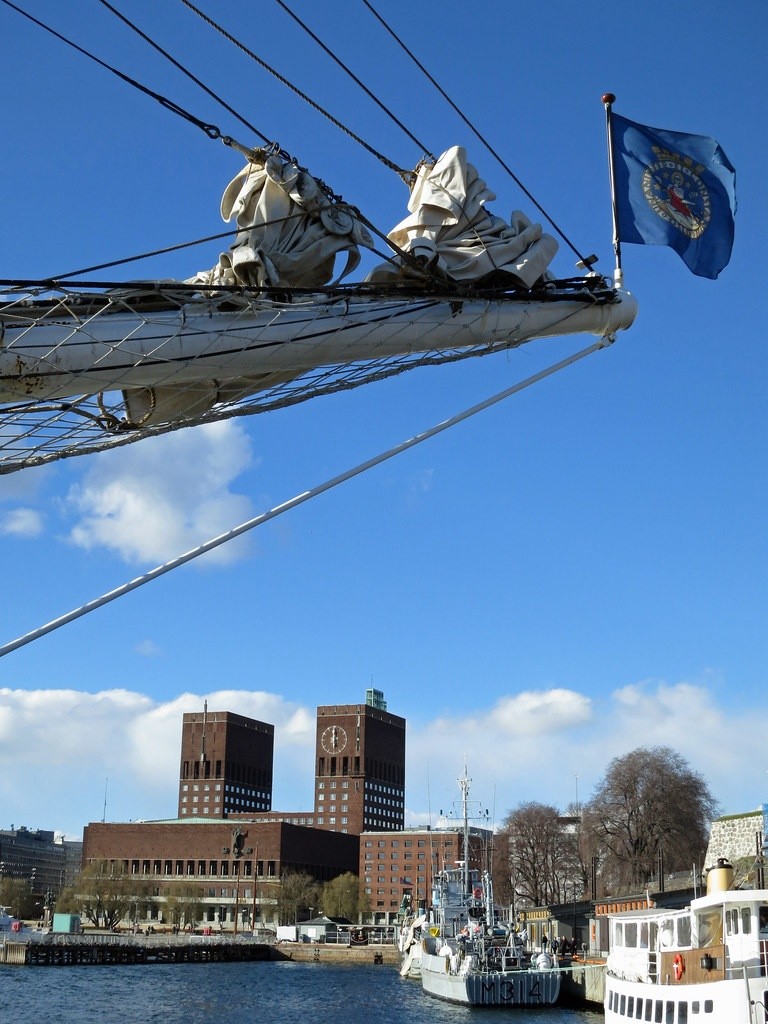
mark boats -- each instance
[603,856,768,1024]
[419,750,561,1007]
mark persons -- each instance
[570,938,578,956]
[560,935,569,956]
[542,934,548,950]
[552,937,559,954]
[112,924,213,937]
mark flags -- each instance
[606,111,738,281]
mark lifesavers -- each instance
[672,953,684,980]
[473,888,485,899]
